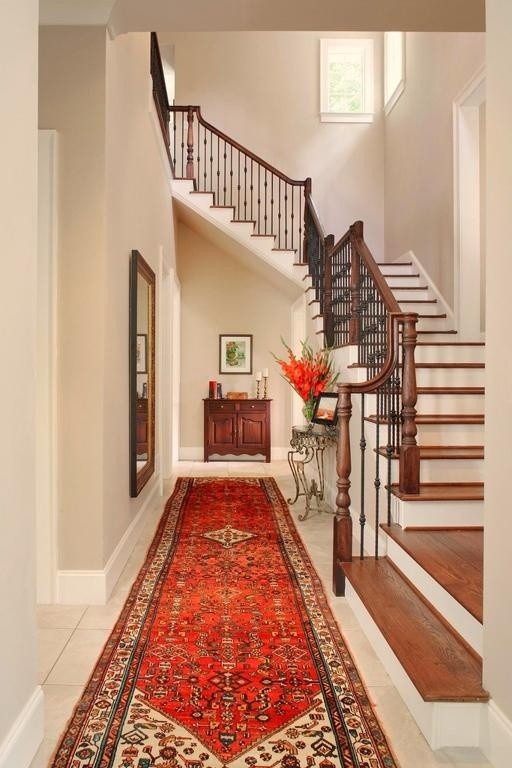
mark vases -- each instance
[301,400,315,429]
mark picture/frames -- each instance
[136,333,147,374]
[318,38,376,121]
[312,391,339,426]
[218,333,254,375]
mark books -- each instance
[227,392,248,399]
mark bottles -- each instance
[209,381,222,400]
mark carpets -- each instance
[51,477,400,767]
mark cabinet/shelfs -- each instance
[203,397,272,463]
[136,399,149,461]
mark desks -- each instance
[286,424,338,521]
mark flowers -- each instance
[267,334,340,419]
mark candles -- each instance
[263,368,269,377]
[256,371,262,381]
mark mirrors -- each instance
[130,250,155,498]
